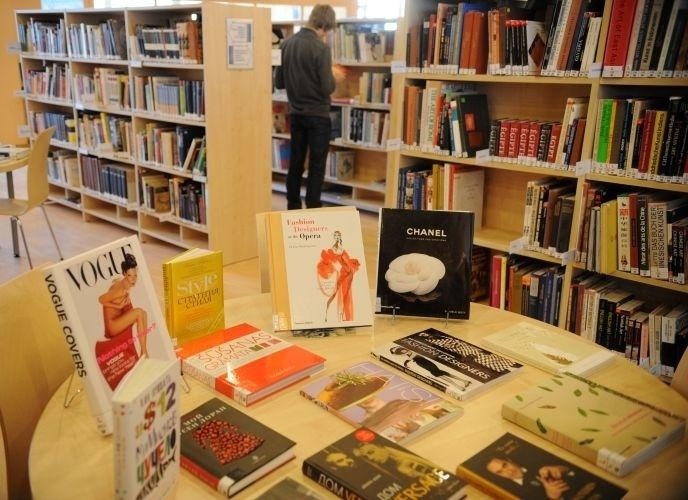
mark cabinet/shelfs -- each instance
[269,13,409,216]
[379,1,688,388]
[2,2,275,268]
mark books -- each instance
[398,161,577,326]
[174,322,327,407]
[173,396,297,498]
[566,184,687,385]
[402,79,687,184]
[502,372,687,478]
[111,354,182,500]
[162,245,225,350]
[371,204,475,322]
[67,19,138,209]
[270,24,391,182]
[130,19,206,226]
[364,328,522,401]
[479,321,601,376]
[41,234,181,435]
[18,17,82,206]
[299,360,464,445]
[265,206,374,331]
[302,426,467,500]
[453,431,628,500]
[405,0,687,78]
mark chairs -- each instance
[0,125,64,270]
[669,346,688,401]
[0,260,78,500]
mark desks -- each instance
[27,290,688,500]
[0,157,28,258]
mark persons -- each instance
[324,452,388,498]
[98,252,150,359]
[360,440,434,480]
[274,4,349,211]
[315,230,362,321]
[484,452,574,499]
